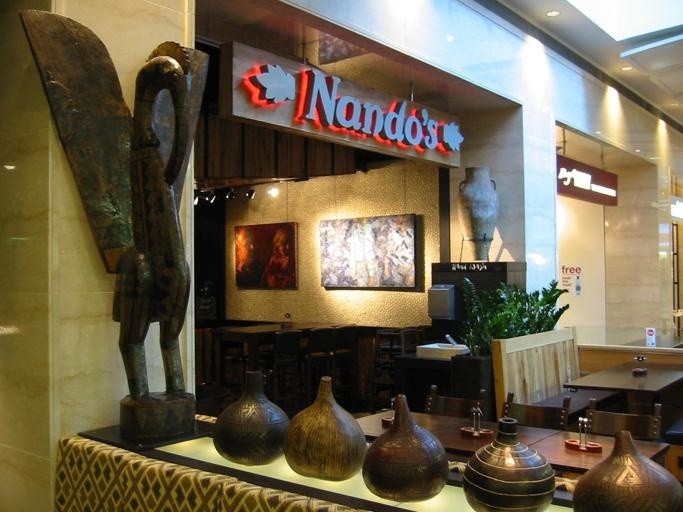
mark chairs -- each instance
[214,322,362,412]
[421,384,664,441]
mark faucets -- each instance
[445,334,457,345]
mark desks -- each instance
[345,410,672,486]
[563,358,683,417]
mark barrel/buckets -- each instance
[416,343,470,362]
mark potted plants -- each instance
[450,273,572,394]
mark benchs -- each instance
[489,327,619,427]
